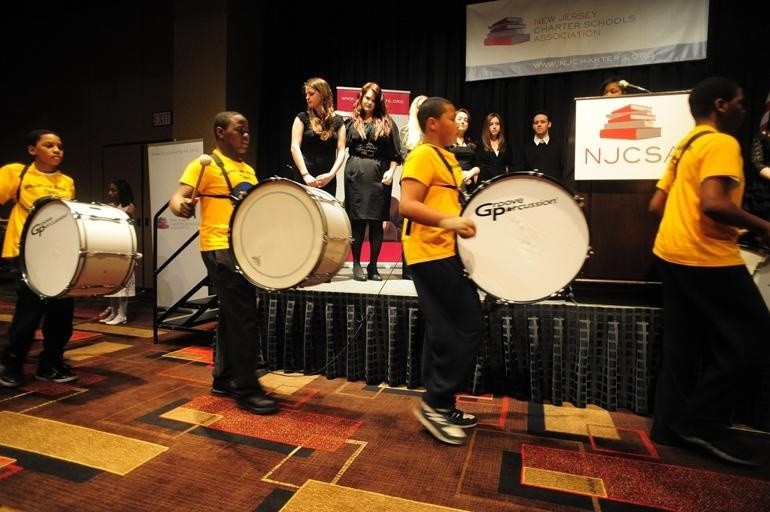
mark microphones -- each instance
[618,80,649,93]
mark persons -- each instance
[289,79,347,196]
[98,181,137,327]
[750,129,770,182]
[403,79,650,194]
[341,83,400,280]
[1,128,80,388]
[648,76,768,443]
[399,98,477,446]
[169,111,278,414]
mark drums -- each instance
[228,178,353,292]
[457,170,593,305]
[19,196,142,301]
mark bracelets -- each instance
[300,172,311,177]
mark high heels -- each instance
[353,267,367,281]
[367,264,383,280]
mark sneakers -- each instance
[411,399,468,445]
[0,354,23,387]
[681,427,767,465]
[449,410,479,429]
[35,351,78,383]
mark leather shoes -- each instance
[238,390,279,414]
[211,382,239,397]
[99,313,128,324]
